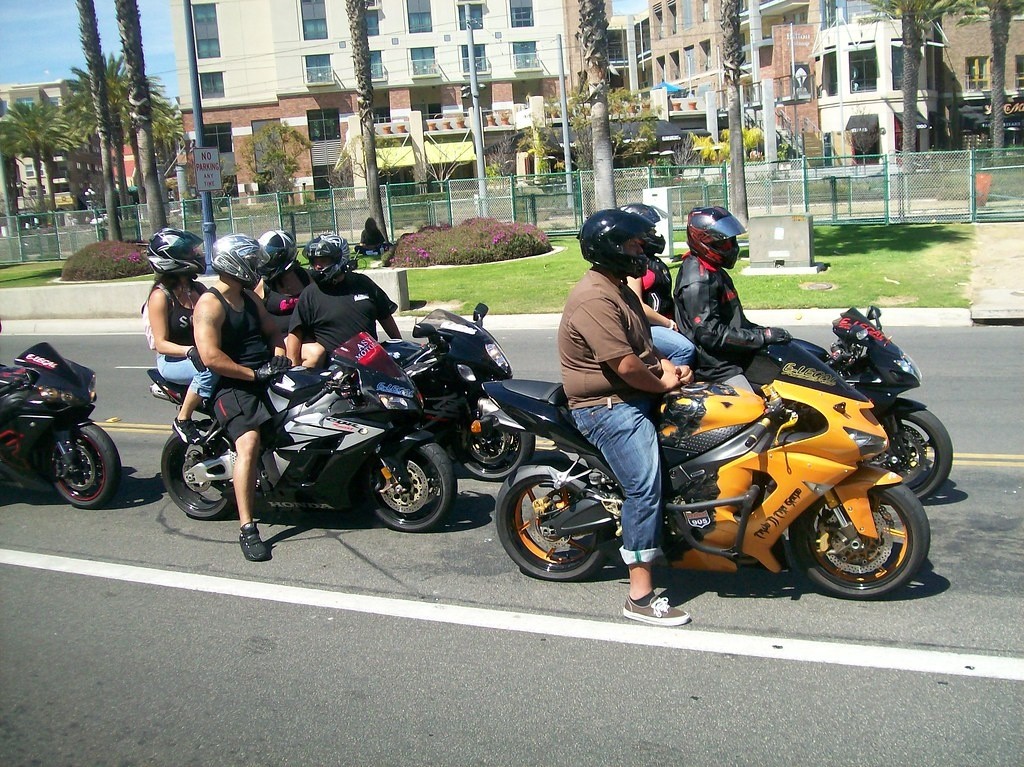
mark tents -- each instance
[362,140,477,171]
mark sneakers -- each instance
[172,417,203,445]
[624,595,689,625]
[239,522,270,562]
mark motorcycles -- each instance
[744,305,954,504]
[147,328,458,534]
[470,335,932,602]
[0,341,122,510]
[376,302,537,482]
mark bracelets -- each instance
[667,319,674,330]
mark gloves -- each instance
[272,353,292,369]
[764,328,792,343]
[253,360,281,379]
[186,348,207,373]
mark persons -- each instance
[146,227,403,562]
[354,217,393,257]
[616,204,695,367]
[558,207,691,626]
[674,206,793,398]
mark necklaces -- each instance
[172,286,195,309]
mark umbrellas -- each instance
[650,81,686,93]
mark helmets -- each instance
[578,212,656,278]
[688,206,744,269]
[211,234,268,292]
[303,232,350,287]
[258,231,297,283]
[619,203,666,254]
[147,229,207,276]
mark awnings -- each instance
[930,112,951,124]
[845,113,878,132]
[808,47,837,59]
[843,41,877,52]
[955,97,1024,128]
[894,110,932,131]
[890,40,926,48]
[926,40,950,48]
